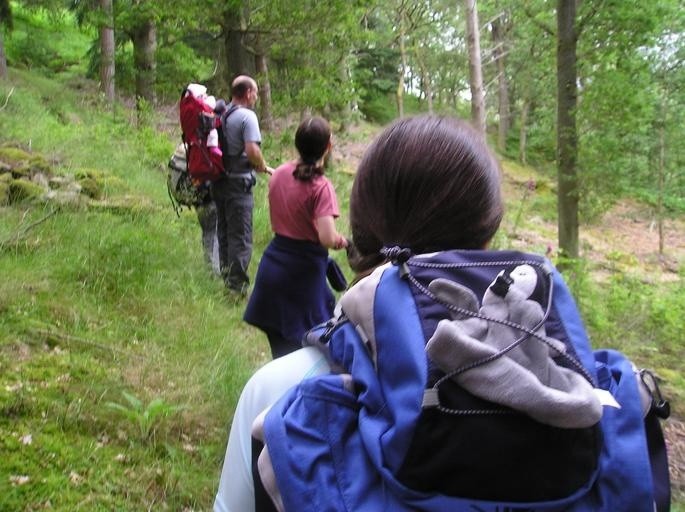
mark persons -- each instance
[196,99,227,275]
[242,116,353,359]
[214,76,266,304]
[211,114,671,512]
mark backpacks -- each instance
[252,243,654,508]
[166,143,214,218]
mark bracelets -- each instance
[335,234,348,251]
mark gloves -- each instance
[426,264,605,430]
